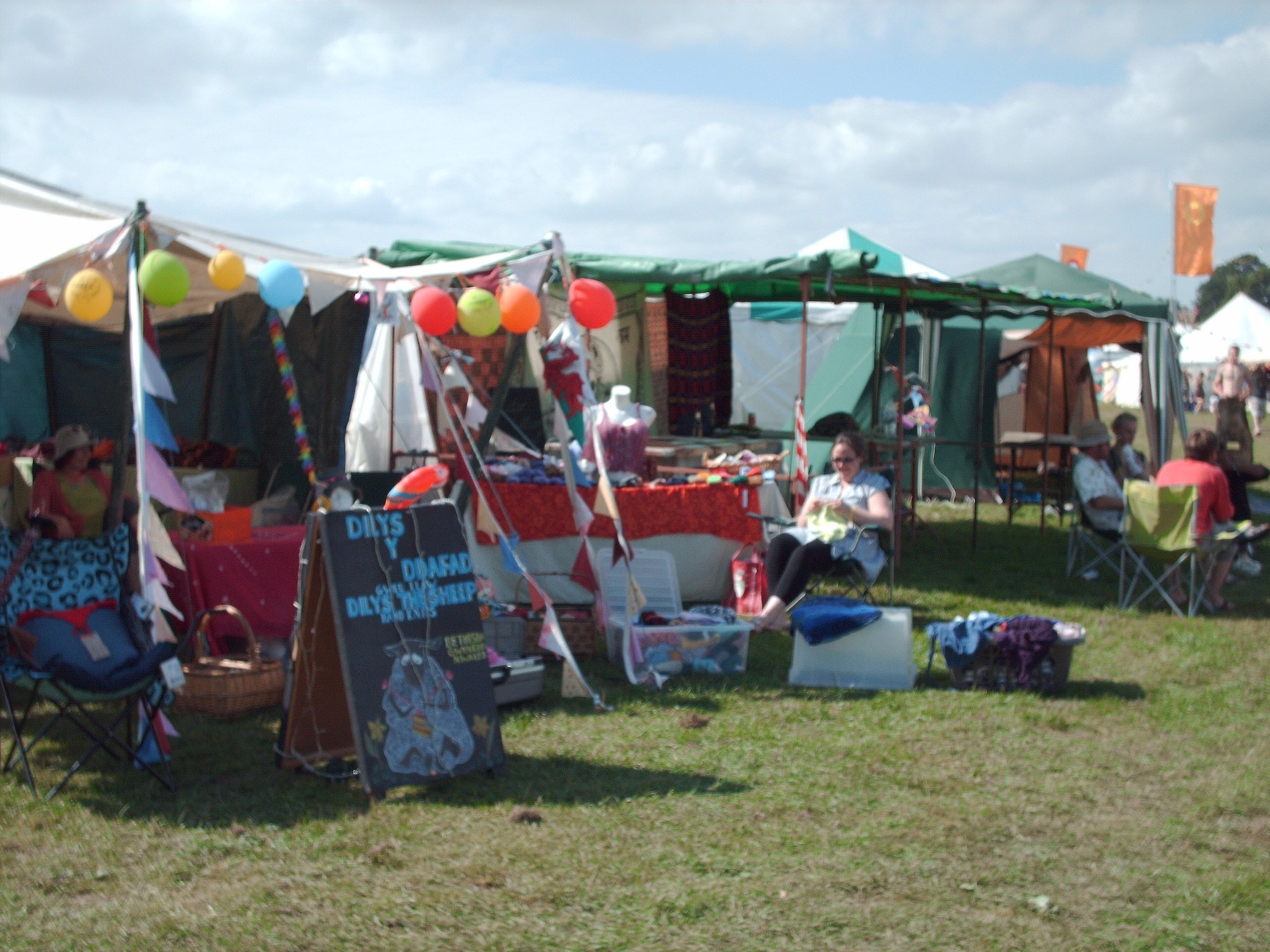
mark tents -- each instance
[0,225,1270,766]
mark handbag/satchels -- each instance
[0,522,130,683]
[18,598,137,683]
[722,540,768,615]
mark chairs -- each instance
[745,465,899,614]
[1066,454,1253,618]
[0,521,228,804]
[265,460,315,525]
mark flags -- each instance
[1173,183,1215,277]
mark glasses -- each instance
[830,456,857,464]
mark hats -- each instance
[1072,420,1113,448]
[52,424,99,462]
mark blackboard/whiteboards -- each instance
[278,503,508,792]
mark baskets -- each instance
[171,605,283,718]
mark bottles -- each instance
[1120,441,1143,478]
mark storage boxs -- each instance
[609,612,755,678]
[788,607,917,690]
[945,620,1084,690]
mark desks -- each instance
[156,524,307,656]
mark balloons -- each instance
[410,286,456,335]
[495,280,542,334]
[207,249,245,290]
[258,258,306,309]
[137,248,191,306]
[453,287,502,338]
[568,276,616,329]
[64,266,114,324]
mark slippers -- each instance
[1219,600,1236,610]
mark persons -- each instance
[1213,345,1254,470]
[30,422,142,597]
[1073,420,1125,539]
[742,430,896,627]
[1106,411,1263,576]
[580,382,656,486]
[1155,426,1238,612]
